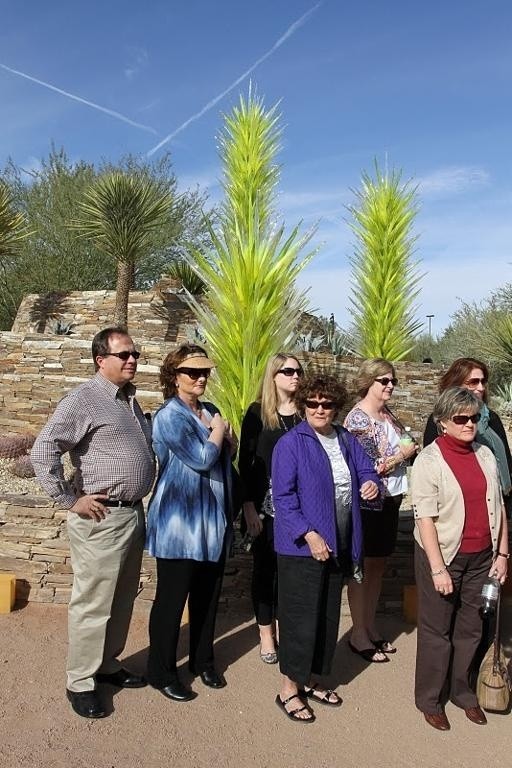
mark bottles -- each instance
[478,568,499,620]
[400,425,414,467]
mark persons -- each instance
[237,352,309,666]
[144,344,239,701]
[271,377,388,723]
[29,327,158,718]
[340,357,419,661]
[410,386,510,730]
[423,357,512,686]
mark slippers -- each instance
[275,692,315,721]
[301,677,345,706]
[347,632,397,664]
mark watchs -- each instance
[497,551,510,560]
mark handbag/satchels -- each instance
[230,463,245,521]
[404,439,417,467]
[475,637,511,714]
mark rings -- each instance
[93,507,99,512]
[316,556,321,560]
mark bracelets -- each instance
[211,422,223,429]
[398,449,408,462]
[429,566,447,576]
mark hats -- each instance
[174,351,215,370]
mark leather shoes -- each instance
[258,638,281,664]
[195,663,228,690]
[64,685,106,719]
[454,693,487,726]
[423,702,452,731]
[97,663,147,688]
[151,675,197,700]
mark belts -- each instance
[98,496,146,507]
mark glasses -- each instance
[174,368,210,379]
[447,412,482,425]
[99,351,141,360]
[465,377,488,388]
[304,400,338,409]
[276,367,303,377]
[374,377,398,385]
